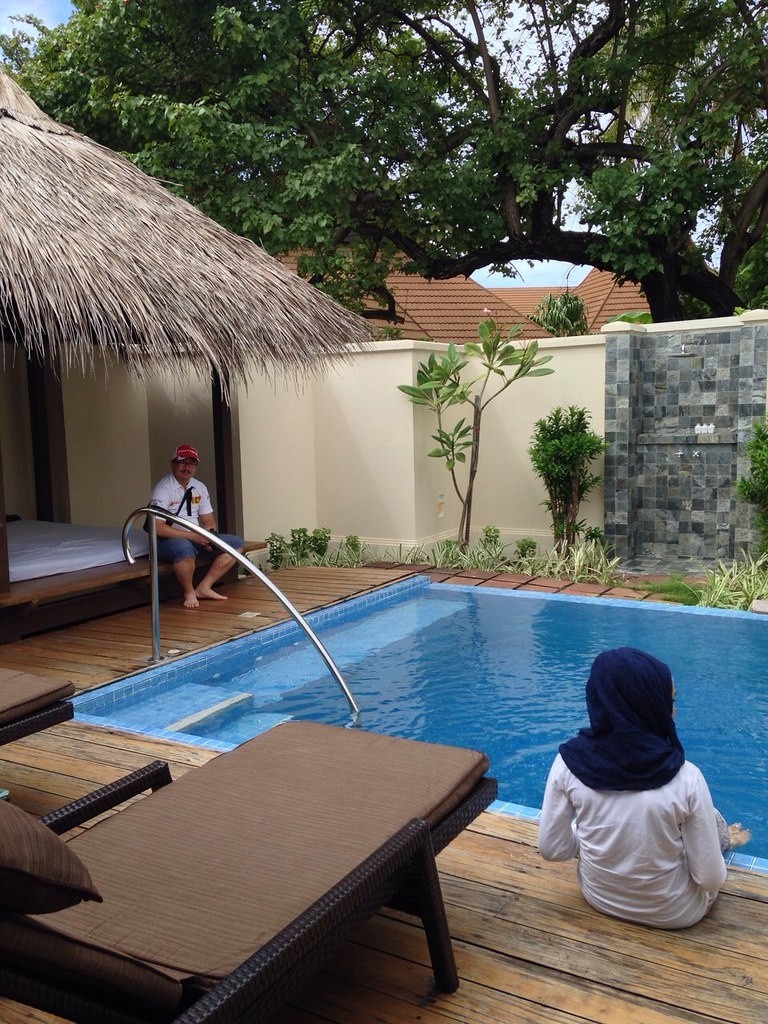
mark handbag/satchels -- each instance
[143,513,173,539]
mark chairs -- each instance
[0,720,498,1023]
[0,665,76,747]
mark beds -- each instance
[6,519,150,583]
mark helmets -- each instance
[170,445,200,464]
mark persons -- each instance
[538,649,753,927]
[151,445,244,607]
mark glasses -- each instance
[173,460,197,467]
